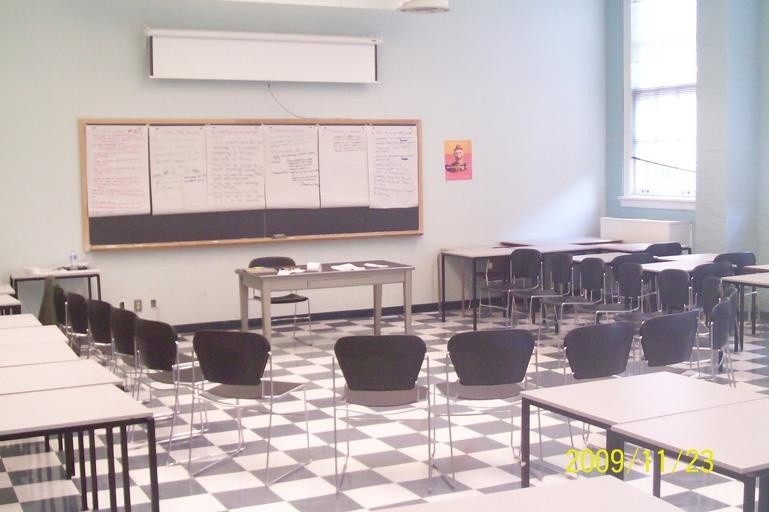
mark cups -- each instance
[306,261,322,273]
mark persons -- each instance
[445,144,468,172]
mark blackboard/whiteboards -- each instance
[77,117,425,254]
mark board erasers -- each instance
[272,233,286,239]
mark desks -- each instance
[235,260,416,342]
[9,266,101,313]
[520,370,768,492]
[372,476,684,511]
[611,396,768,511]
[1,383,160,512]
[1,283,143,512]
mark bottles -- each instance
[69,250,79,272]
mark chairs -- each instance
[431,328,543,489]
[127,318,247,466]
[538,300,739,471]
[187,330,311,487]
[249,257,312,347]
[332,335,433,494]
[438,236,768,353]
